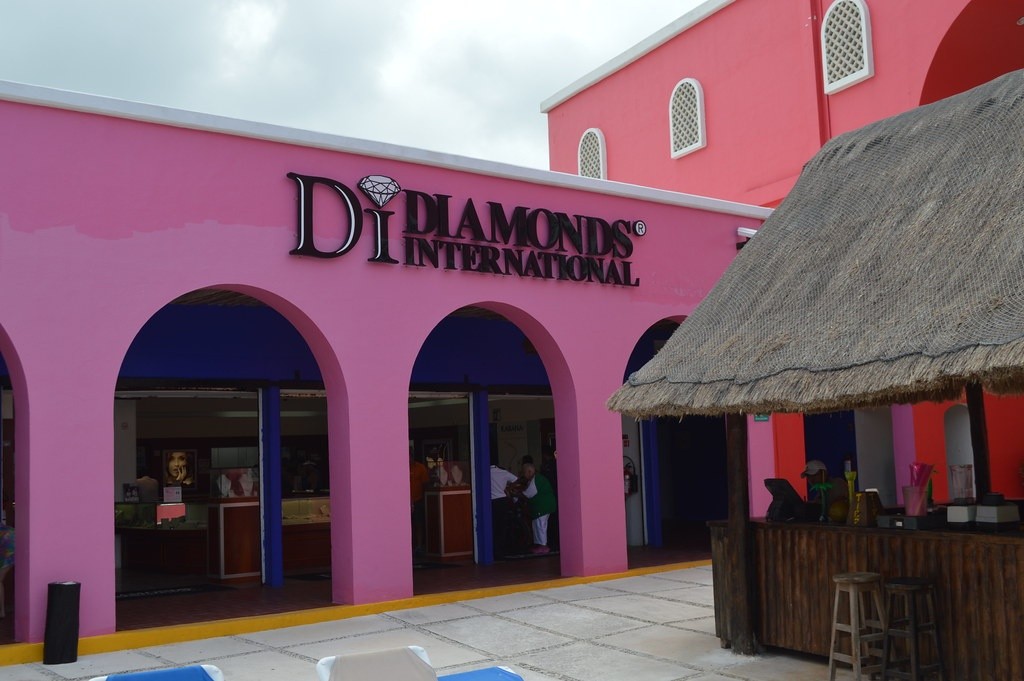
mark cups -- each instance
[902,486,927,516]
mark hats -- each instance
[801,460,826,477]
[521,454,534,465]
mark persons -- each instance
[0,520,15,619]
[137,465,159,503]
[163,450,202,505]
[515,461,556,555]
[492,454,523,558]
[410,445,429,570]
[801,461,848,521]
[542,445,556,483]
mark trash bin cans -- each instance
[43,580,81,664]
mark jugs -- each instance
[949,464,973,499]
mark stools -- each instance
[881,576,947,681]
[826,571,901,681]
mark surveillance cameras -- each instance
[737,227,757,238]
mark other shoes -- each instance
[531,545,549,553]
